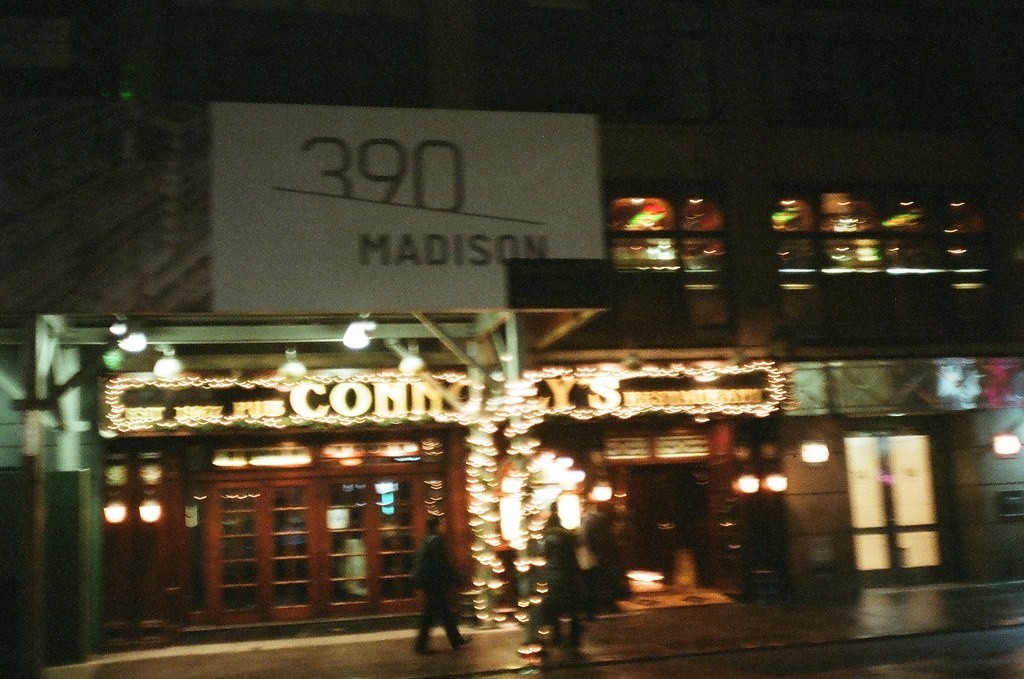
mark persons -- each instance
[538,503,589,644]
[585,505,630,618]
[411,516,476,655]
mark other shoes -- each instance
[413,646,430,654]
[452,635,472,648]
